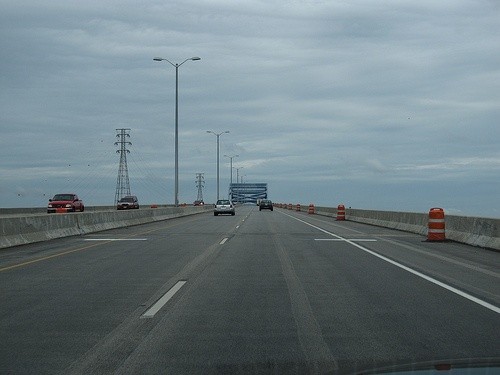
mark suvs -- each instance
[257,198,273,212]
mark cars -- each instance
[193,200,204,206]
[47,194,85,212]
[212,200,236,216]
[117,196,139,210]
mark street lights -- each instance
[207,130,230,201]
[153,57,201,207]
[224,154,248,205]
